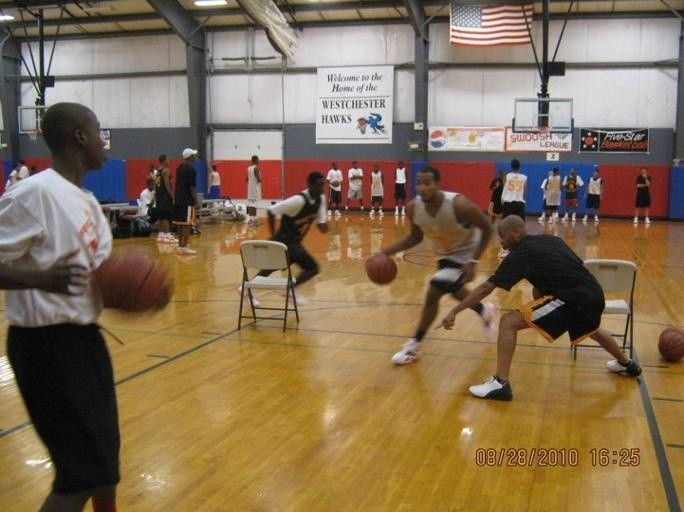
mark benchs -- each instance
[100,196,238,237]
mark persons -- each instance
[207,162,224,203]
[631,168,655,226]
[486,157,603,225]
[433,214,643,402]
[236,172,340,307]
[363,164,494,364]
[244,155,266,225]
[138,149,203,255]
[394,162,408,218]
[0,157,37,193]
[0,103,175,511]
[325,160,386,217]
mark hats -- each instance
[182,148,198,160]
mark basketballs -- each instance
[99,249,171,312]
[368,254,397,283]
[659,328,684,361]
[333,180,339,187]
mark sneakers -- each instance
[605,359,641,376]
[391,338,422,365]
[282,288,305,306]
[645,218,650,223]
[237,285,260,309]
[634,218,638,223]
[156,233,197,255]
[483,302,497,335]
[468,376,512,400]
[537,216,599,222]
[328,205,406,216]
[497,248,510,259]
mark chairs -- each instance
[238,240,300,333]
[570,259,637,361]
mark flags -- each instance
[448,1,532,46]
